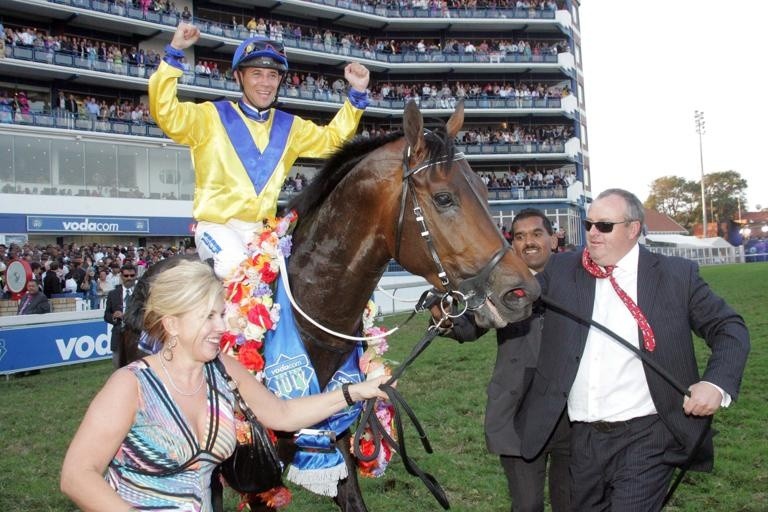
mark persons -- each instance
[552,222,566,252]
[362,0,557,13]
[479,168,576,190]
[0,241,42,300]
[370,81,571,102]
[149,23,369,283]
[15,280,51,377]
[281,172,307,193]
[465,125,577,150]
[285,25,363,56]
[205,17,283,41]
[372,37,571,57]
[97,271,115,309]
[104,265,142,368]
[43,262,62,299]
[497,222,514,246]
[1,90,151,121]
[424,188,749,510]
[84,266,100,310]
[177,58,220,79]
[0,22,160,79]
[106,264,122,289]
[429,207,559,511]
[107,0,191,22]
[42,243,197,274]
[65,262,86,293]
[59,260,397,511]
[281,71,346,93]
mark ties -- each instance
[582,247,656,351]
[123,291,130,323]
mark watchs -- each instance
[342,382,356,406]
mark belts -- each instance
[590,420,631,433]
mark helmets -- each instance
[231,37,288,74]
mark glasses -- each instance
[123,274,136,277]
[583,220,632,234]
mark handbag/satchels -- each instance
[213,357,281,492]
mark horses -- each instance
[116,98,542,511]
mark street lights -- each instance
[693,108,710,241]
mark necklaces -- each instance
[160,352,208,397]
